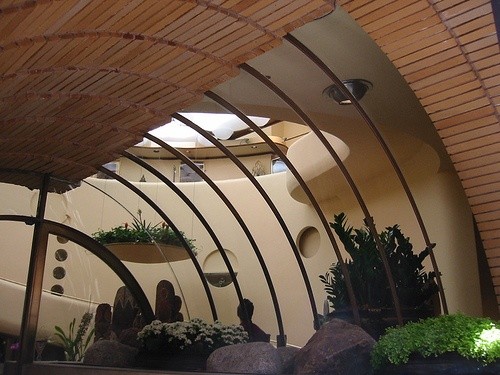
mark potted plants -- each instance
[90,207,199,264]
